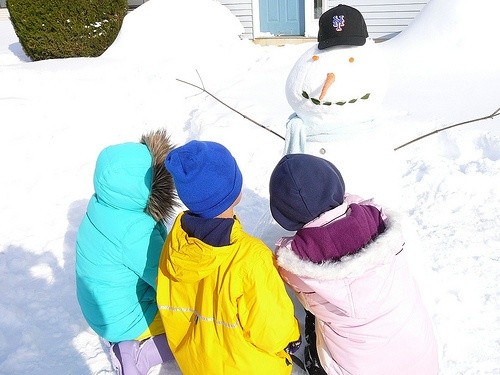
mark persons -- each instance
[156,140,307,375]
[75,129,182,375]
[269,154,440,375]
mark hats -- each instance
[163,139,242,217]
[317,4,367,50]
